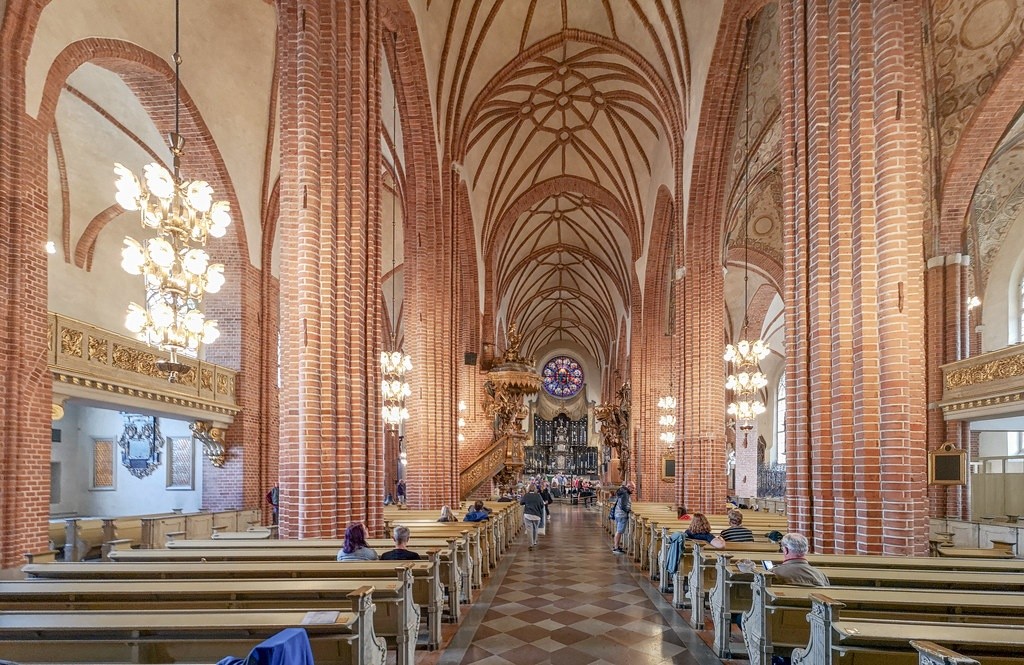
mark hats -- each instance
[765,530,783,542]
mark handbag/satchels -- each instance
[608,507,615,520]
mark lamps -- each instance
[723,20,769,434]
[658,305,676,452]
[114,0,232,382]
[380,32,413,438]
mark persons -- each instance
[379,525,420,560]
[613,480,635,554]
[397,479,406,504]
[498,474,600,551]
[266,482,279,525]
[337,522,378,562]
[684,513,726,549]
[463,500,490,522]
[436,505,458,522]
[735,532,831,665]
[727,496,737,505]
[720,508,755,542]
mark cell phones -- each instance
[763,560,773,570]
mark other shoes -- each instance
[528,545,534,549]
[533,542,537,544]
[613,546,625,554]
[546,515,551,520]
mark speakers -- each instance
[464,353,477,365]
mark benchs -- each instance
[0,498,525,665]
[601,498,1024,665]
